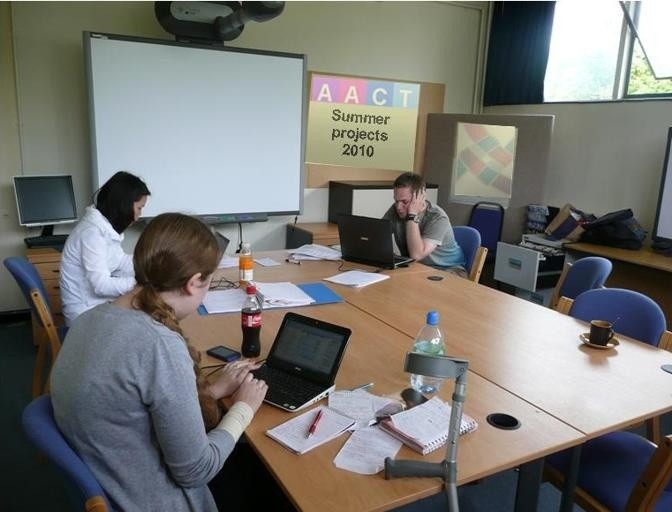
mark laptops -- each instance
[335,212,415,269]
[12,173,79,248]
[215,230,230,264]
[248,312,352,413]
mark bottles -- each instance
[410,311,446,394]
[240,285,262,358]
[238,242,254,287]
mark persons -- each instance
[380,171,468,280]
[59,172,150,328]
[49,213,268,512]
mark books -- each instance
[265,404,357,456]
[379,396,479,456]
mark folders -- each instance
[198,282,343,315]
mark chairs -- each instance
[175,252,586,512]
[452,225,488,284]
[21,394,114,512]
[550,256,612,310]
[468,200,504,278]
[3,257,70,402]
[553,288,672,443]
[541,431,672,512]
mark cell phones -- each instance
[206,346,241,362]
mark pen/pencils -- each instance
[353,383,374,392]
[286,259,303,265]
[308,410,322,438]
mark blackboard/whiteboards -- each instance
[11,2,485,217]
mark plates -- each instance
[580,333,620,349]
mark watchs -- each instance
[407,216,419,223]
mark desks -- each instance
[274,245,672,512]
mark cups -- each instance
[589,320,615,345]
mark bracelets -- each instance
[408,214,415,216]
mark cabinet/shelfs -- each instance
[27,244,67,346]
[552,242,672,335]
[286,222,340,248]
[329,179,438,225]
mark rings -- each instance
[234,364,240,369]
